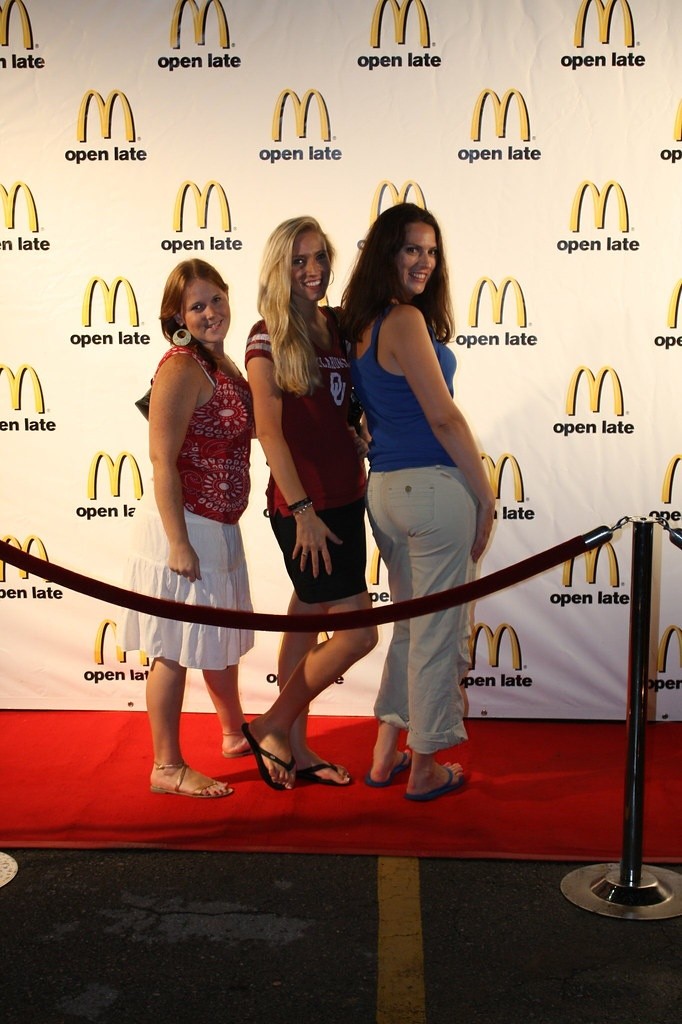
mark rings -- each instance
[301,552,307,555]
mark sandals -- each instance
[150,761,233,797]
[222,732,254,757]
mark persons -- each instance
[240,215,379,790]
[339,203,495,801]
[115,257,254,798]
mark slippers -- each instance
[241,722,296,790]
[404,766,463,801]
[365,752,411,787]
[295,761,352,786]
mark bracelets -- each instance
[287,496,312,516]
